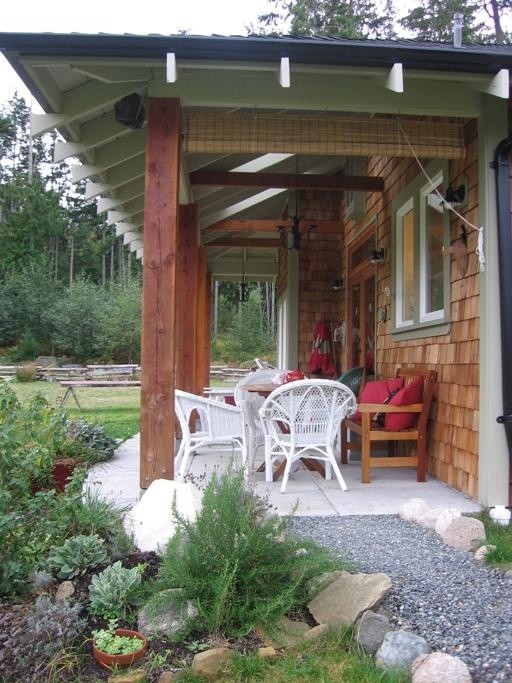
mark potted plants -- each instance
[92,616,150,673]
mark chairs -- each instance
[173,367,375,494]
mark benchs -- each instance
[342,367,437,484]
[37,364,141,410]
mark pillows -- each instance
[353,376,423,432]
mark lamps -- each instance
[370,244,386,264]
[234,247,250,302]
[278,154,317,251]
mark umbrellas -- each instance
[308,314,340,379]
